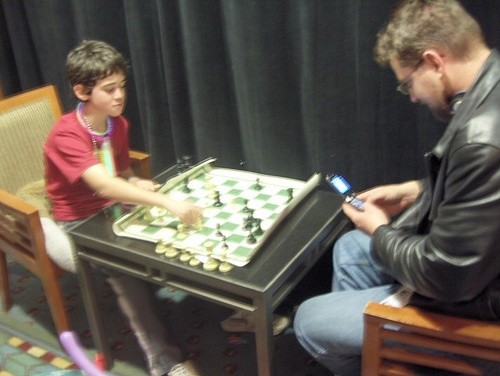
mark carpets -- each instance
[0,324,115,376]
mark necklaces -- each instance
[82,111,112,164]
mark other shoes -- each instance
[167,359,198,376]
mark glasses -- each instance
[395,54,445,95]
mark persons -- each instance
[294,1,500,376]
[43,39,202,375]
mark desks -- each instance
[67,164,355,376]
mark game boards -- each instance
[113,158,321,267]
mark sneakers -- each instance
[220,306,290,337]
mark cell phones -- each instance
[326,172,365,209]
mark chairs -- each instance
[362,303,500,376]
[0,83,153,337]
